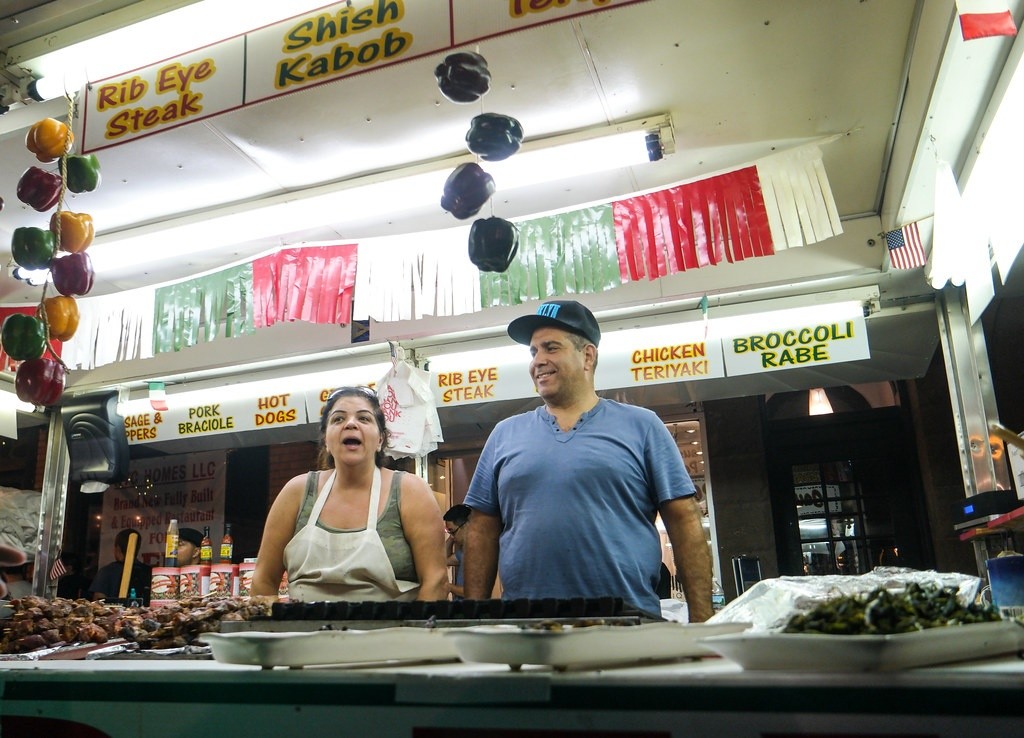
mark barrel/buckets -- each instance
[984,555,1024,606]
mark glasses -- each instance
[327,386,377,401]
[448,520,467,536]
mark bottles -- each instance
[200,526,213,566]
[220,523,233,564]
[164,518,179,567]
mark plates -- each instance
[198,602,1023,671]
[151,569,254,597]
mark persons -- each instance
[460,299,717,628]
[251,384,449,603]
[59,551,98,599]
[442,505,473,603]
[175,528,202,566]
[93,529,151,604]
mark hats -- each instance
[507,300,601,347]
[179,528,204,547]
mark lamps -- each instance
[8,112,678,287]
